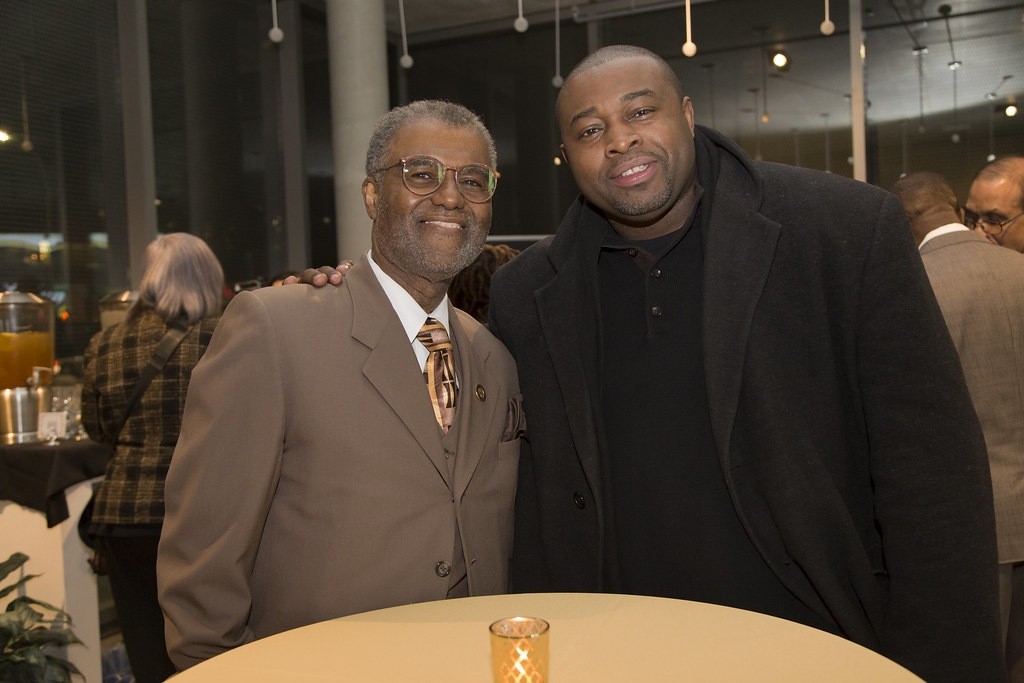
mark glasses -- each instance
[372,153,502,203]
[960,210,1024,236]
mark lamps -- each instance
[938,4,962,70]
[890,0,929,56]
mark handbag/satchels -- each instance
[78,490,101,550]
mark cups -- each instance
[0,389,38,446]
[51,383,83,433]
[488,616,551,683]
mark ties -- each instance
[416,318,460,435]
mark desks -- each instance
[0,438,114,509]
[159,591,929,683]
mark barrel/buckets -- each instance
[0,291,56,388]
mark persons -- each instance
[78,45,1024,683]
